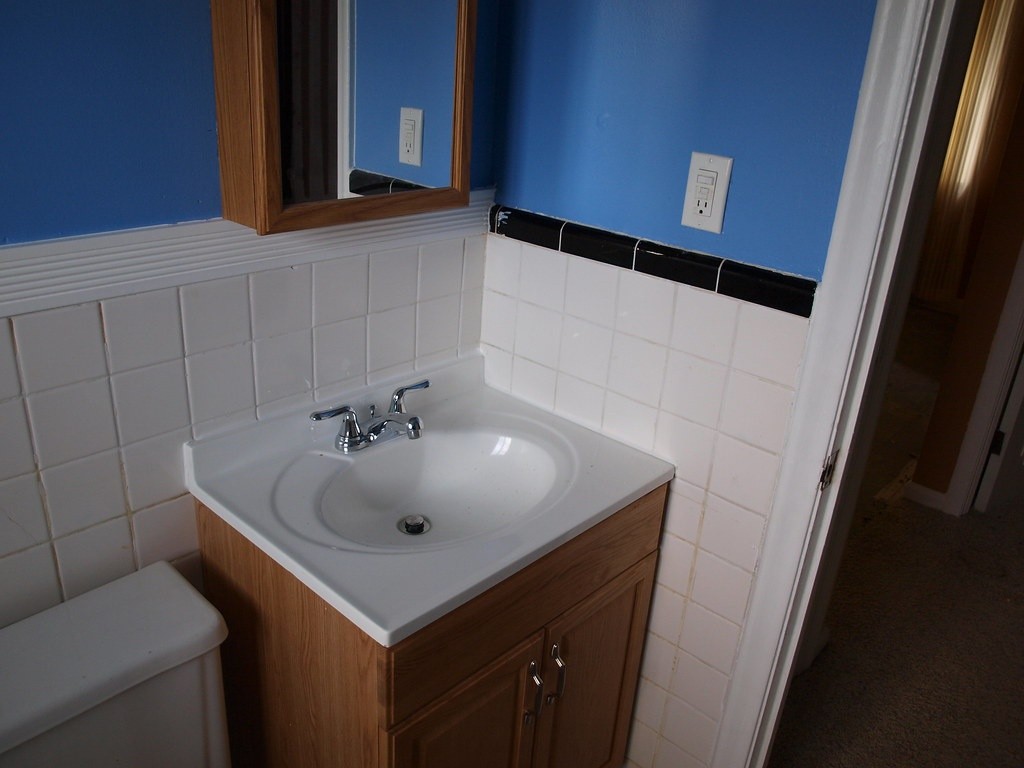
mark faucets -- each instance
[363,412,425,440]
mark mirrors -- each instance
[249,0,471,236]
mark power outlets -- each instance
[397,107,424,166]
[682,151,732,236]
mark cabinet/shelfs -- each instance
[193,481,671,768]
[210,0,470,237]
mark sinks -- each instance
[269,407,581,553]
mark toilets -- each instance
[0,559,233,768]
